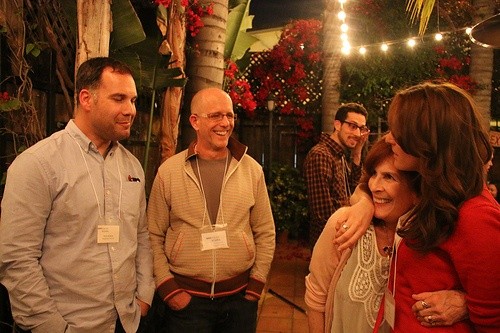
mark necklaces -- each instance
[382,225,394,258]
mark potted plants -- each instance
[266,161,309,243]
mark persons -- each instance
[0,56,155,333]
[304,134,423,333]
[333,83,500,333]
[147,87,276,333]
[305,103,370,257]
[483,148,498,198]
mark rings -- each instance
[421,300,429,308]
[428,316,432,321]
[342,224,348,230]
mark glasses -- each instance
[340,120,368,134]
[197,113,237,122]
